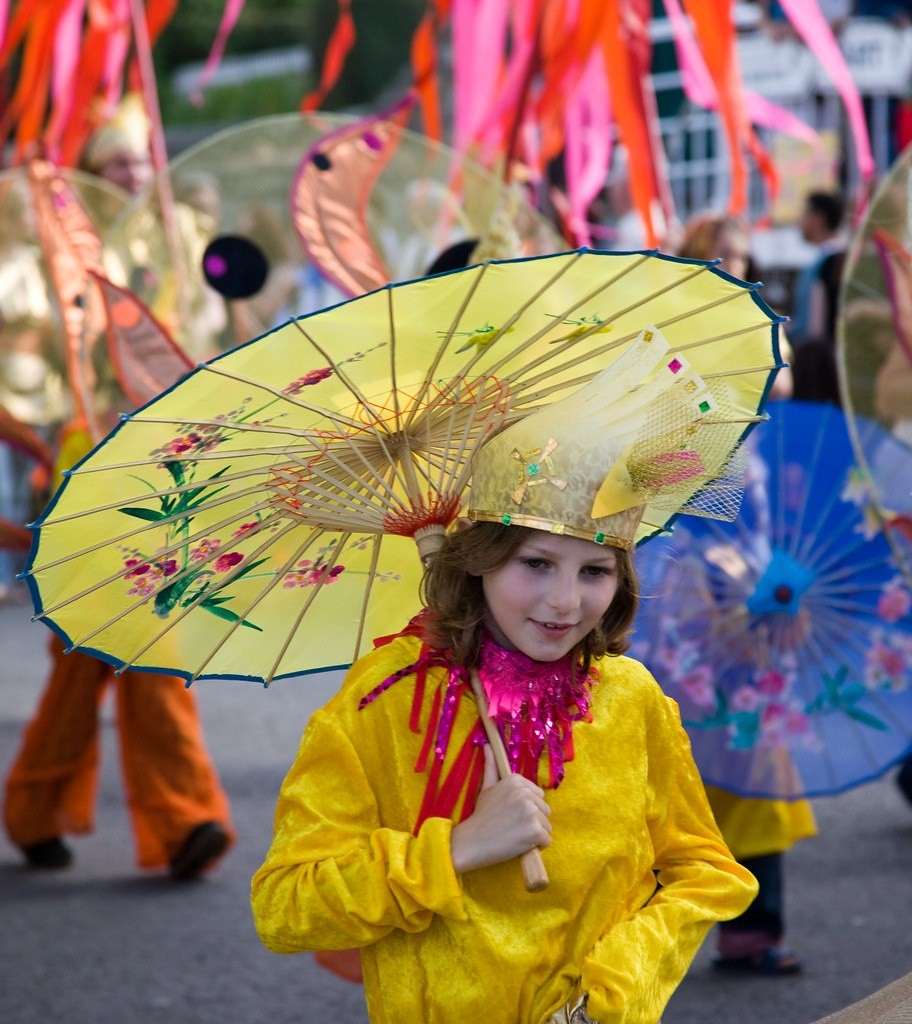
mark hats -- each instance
[468,324,750,554]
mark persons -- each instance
[651,454,796,972]
[801,191,888,417]
[250,412,759,1023]
[424,240,480,277]
[2,326,236,883]
[676,218,792,397]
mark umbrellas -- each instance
[0,114,912,797]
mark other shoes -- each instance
[712,941,803,974]
[169,822,228,884]
[20,838,71,873]
